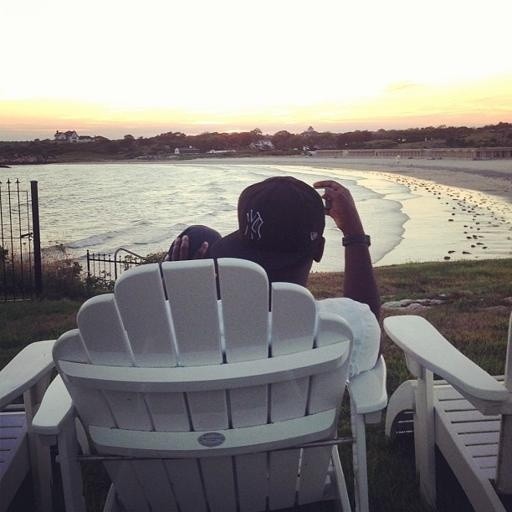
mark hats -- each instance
[210,176,326,270]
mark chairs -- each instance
[383,315,511,510]
[32,260,388,510]
[2,340,56,511]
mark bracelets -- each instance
[342,233,372,247]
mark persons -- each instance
[155,175,385,369]
